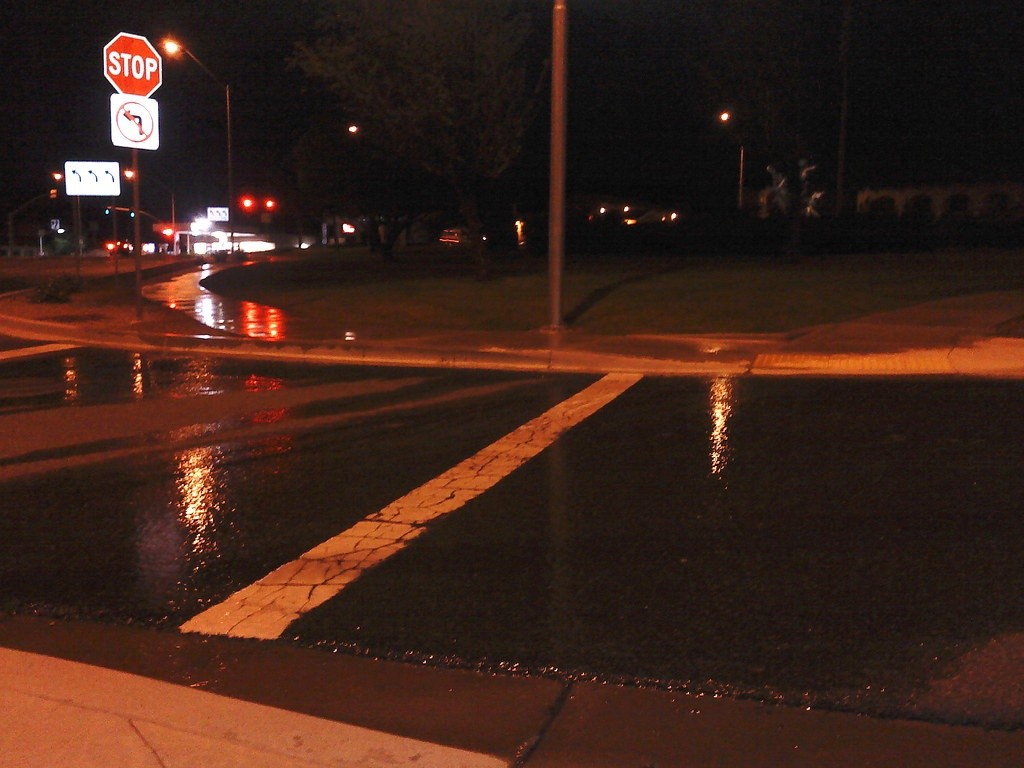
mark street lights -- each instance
[163,38,240,258]
[51,171,82,261]
[348,124,371,250]
[719,111,746,214]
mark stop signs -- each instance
[104,32,164,99]
